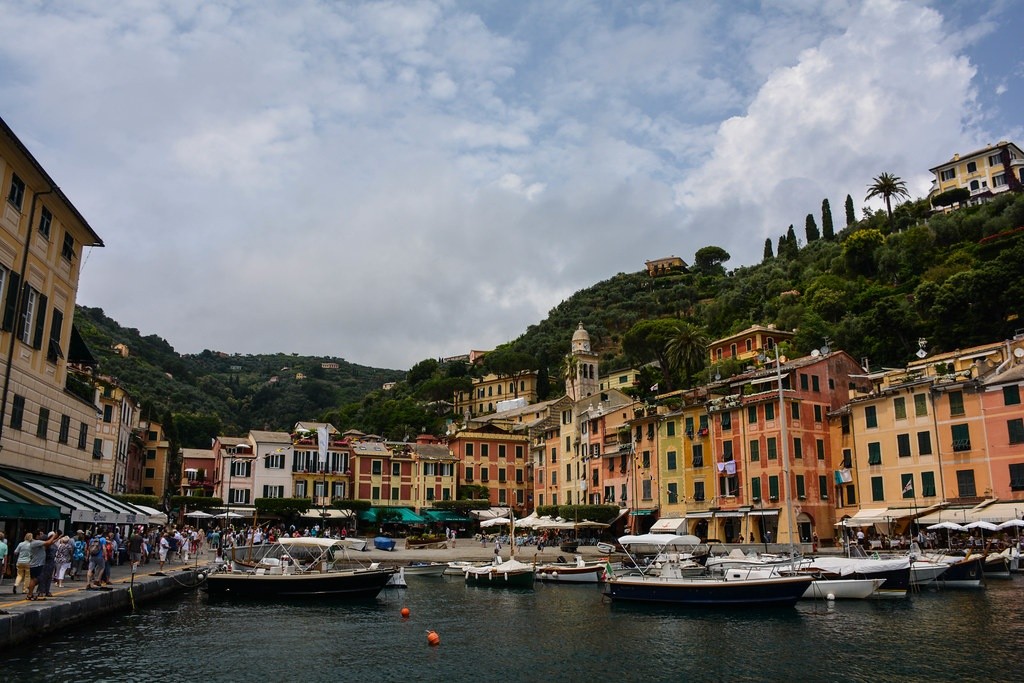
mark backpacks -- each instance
[88,538,101,556]
[73,541,84,560]
[56,545,68,563]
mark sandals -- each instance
[38,593,43,597]
[26,595,38,601]
[46,593,52,597]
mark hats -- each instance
[77,529,84,534]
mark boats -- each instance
[373,536,396,551]
[596,541,617,554]
[393,560,450,578]
[186,485,1024,613]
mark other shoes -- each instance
[53,570,120,588]
[13,585,17,594]
[23,590,28,594]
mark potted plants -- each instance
[365,526,379,538]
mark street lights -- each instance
[224,452,237,551]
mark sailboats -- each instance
[603,346,819,609]
[559,497,581,553]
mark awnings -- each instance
[686,508,782,518]
[299,509,349,518]
[360,507,428,524]
[0,468,151,525]
[0,483,61,549]
[835,502,1024,525]
[491,508,509,517]
[651,519,687,535]
[424,510,474,522]
[468,510,496,520]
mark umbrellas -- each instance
[516,512,545,527]
[213,512,244,523]
[480,517,510,532]
[184,511,213,528]
[926,519,1024,549]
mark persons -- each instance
[812,532,818,550]
[738,531,771,543]
[856,529,937,550]
[0,523,349,614]
[445,526,562,563]
